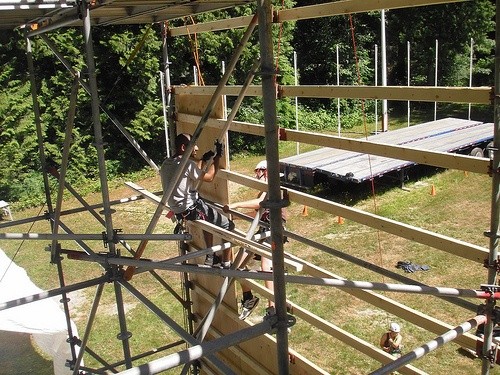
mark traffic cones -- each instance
[337,215,344,226]
[430,184,437,196]
[302,205,308,217]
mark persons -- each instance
[475,306,500,350]
[222,160,291,334]
[381,323,403,359]
[161,134,251,272]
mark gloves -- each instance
[202,150,215,162]
[212,139,224,161]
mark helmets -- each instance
[253,160,266,174]
[389,323,400,333]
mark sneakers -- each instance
[238,295,259,321]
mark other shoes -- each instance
[212,260,232,270]
[204,253,222,267]
